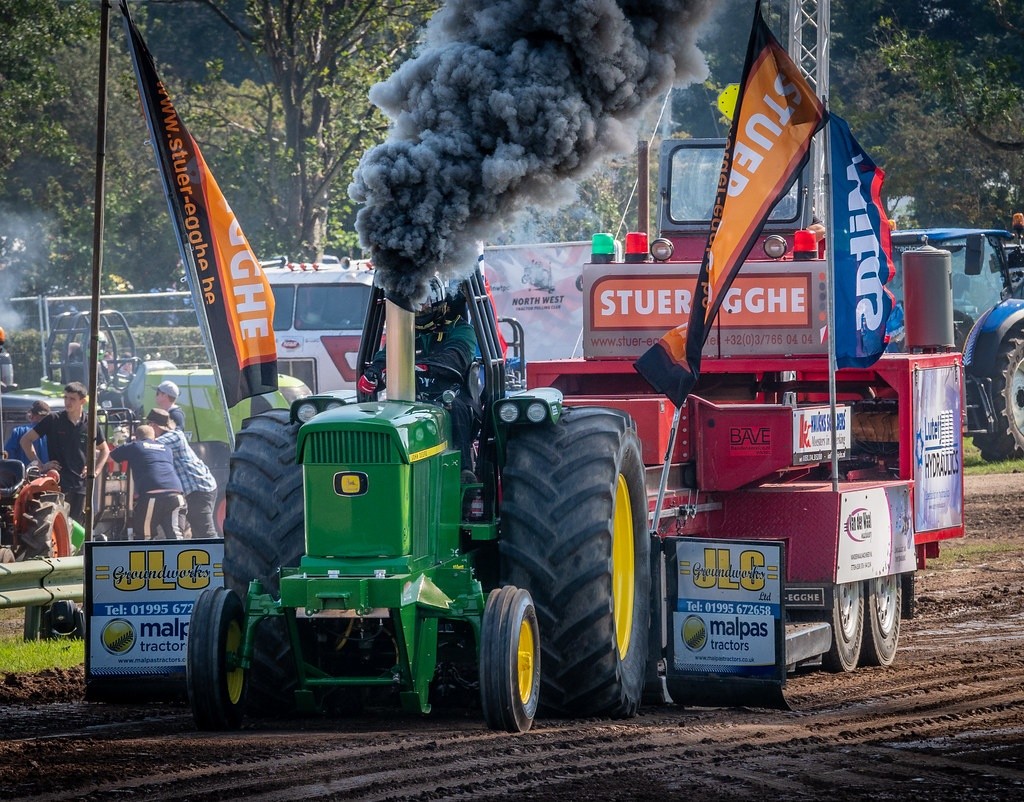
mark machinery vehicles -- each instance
[889,213,1024,465]
[0,302,314,537]
[185,137,964,732]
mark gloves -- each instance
[357,370,379,396]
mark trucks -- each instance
[258,239,623,405]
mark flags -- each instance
[632,7,829,411]
[119,4,278,409]
[829,111,895,370]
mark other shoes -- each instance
[461,471,477,521]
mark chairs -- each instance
[67,342,81,357]
[445,286,468,324]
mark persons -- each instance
[19,382,110,527]
[704,194,826,243]
[60,343,163,403]
[358,275,485,503]
[4,400,51,467]
[106,380,218,540]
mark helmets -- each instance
[81,329,108,362]
[415,276,448,332]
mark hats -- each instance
[142,408,172,431]
[150,380,180,399]
